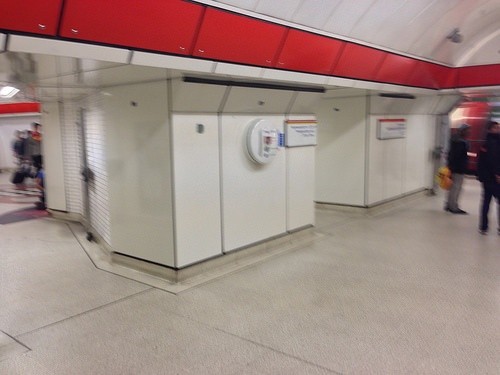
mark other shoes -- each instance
[478,230,487,235]
[447,208,466,214]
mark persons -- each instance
[477,121,500,236]
[12,121,45,209]
[443,123,471,215]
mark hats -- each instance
[451,124,459,129]
[461,124,471,129]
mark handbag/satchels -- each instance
[435,166,453,190]
